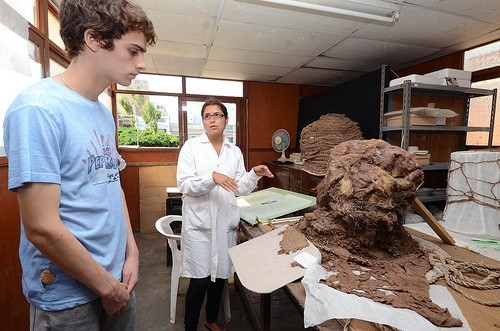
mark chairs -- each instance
[155,215,232,324]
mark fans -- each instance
[272,129,290,162]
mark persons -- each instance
[3,0,159,331]
[177,100,275,331]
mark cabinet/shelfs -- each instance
[380,62,497,221]
[263,161,325,197]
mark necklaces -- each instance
[59,73,99,101]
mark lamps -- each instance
[233,0,400,27]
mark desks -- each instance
[235,197,500,331]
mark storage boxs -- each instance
[423,68,473,88]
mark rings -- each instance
[228,182,231,184]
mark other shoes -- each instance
[204,320,223,331]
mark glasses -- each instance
[202,112,225,120]
